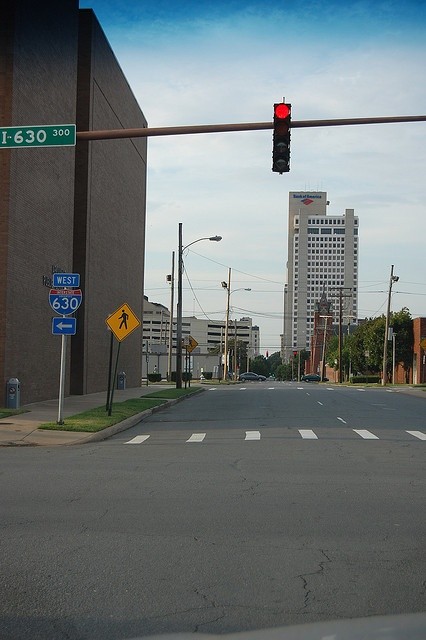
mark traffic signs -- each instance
[53,273,79,288]
[51,318,76,335]
[48,288,80,315]
[0,125,76,149]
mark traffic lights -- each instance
[272,103,291,173]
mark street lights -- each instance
[178,236,223,388]
[220,281,251,380]
[382,276,400,385]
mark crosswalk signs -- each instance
[105,303,140,341]
[182,336,198,352]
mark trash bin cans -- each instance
[118,371,126,390]
[6,378,19,410]
